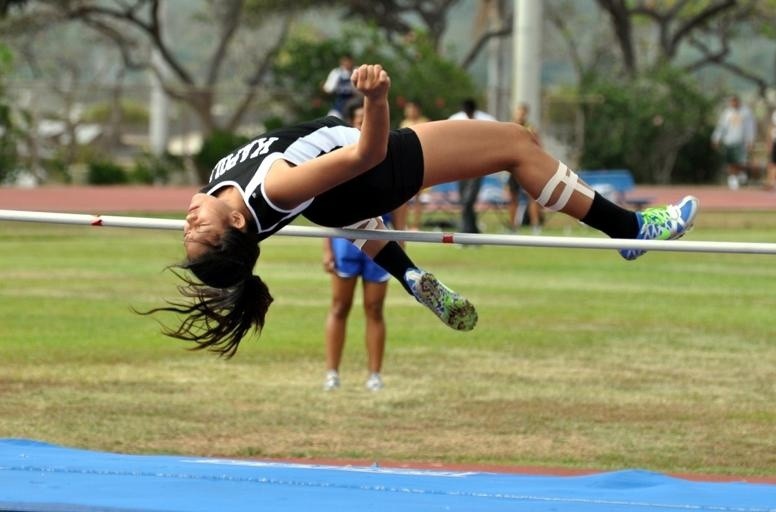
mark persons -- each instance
[442,99,498,248]
[710,94,756,190]
[506,104,545,234]
[319,52,364,110]
[134,63,701,365]
[397,100,434,231]
[318,100,403,389]
[759,110,776,189]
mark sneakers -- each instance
[405,269,477,331]
[619,195,698,259]
[365,374,382,389]
[325,371,341,391]
